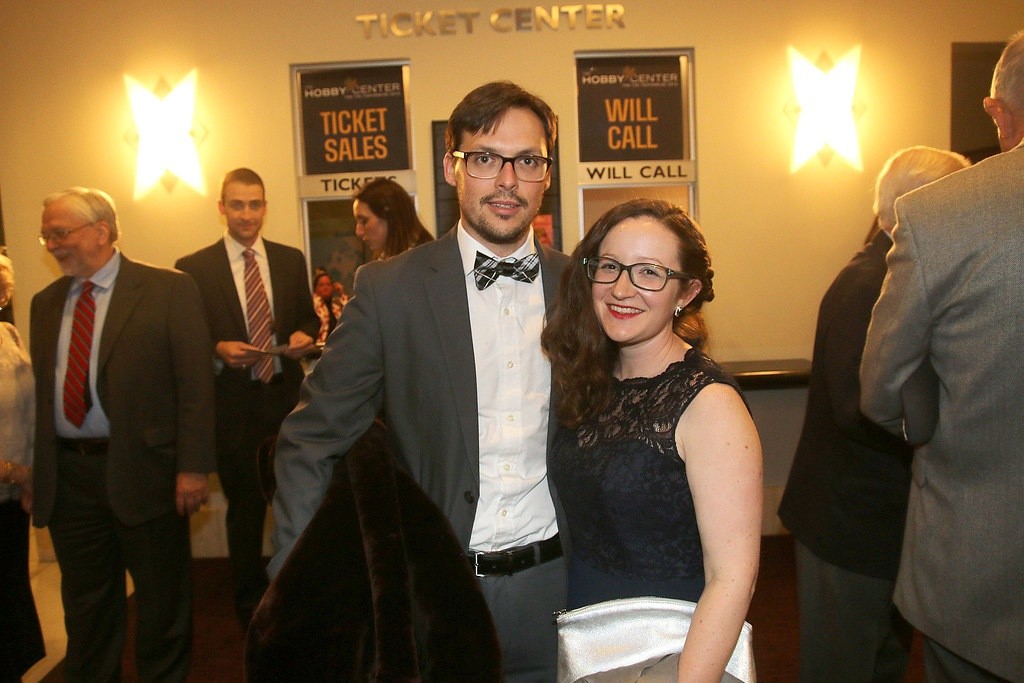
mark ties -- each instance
[242,249,274,384]
[63,282,96,428]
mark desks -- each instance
[717,359,813,390]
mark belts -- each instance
[467,534,563,577]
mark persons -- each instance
[176,167,323,625]
[778,146,973,683]
[351,178,435,259]
[859,29,1024,683]
[540,199,763,683]
[265,80,570,682]
[311,272,348,343]
[0,253,48,683]
[30,189,218,683]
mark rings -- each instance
[242,365,246,369]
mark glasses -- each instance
[452,151,553,183]
[37,221,90,246]
[583,256,697,292]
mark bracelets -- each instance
[1,460,15,485]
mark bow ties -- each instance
[474,251,539,290]
